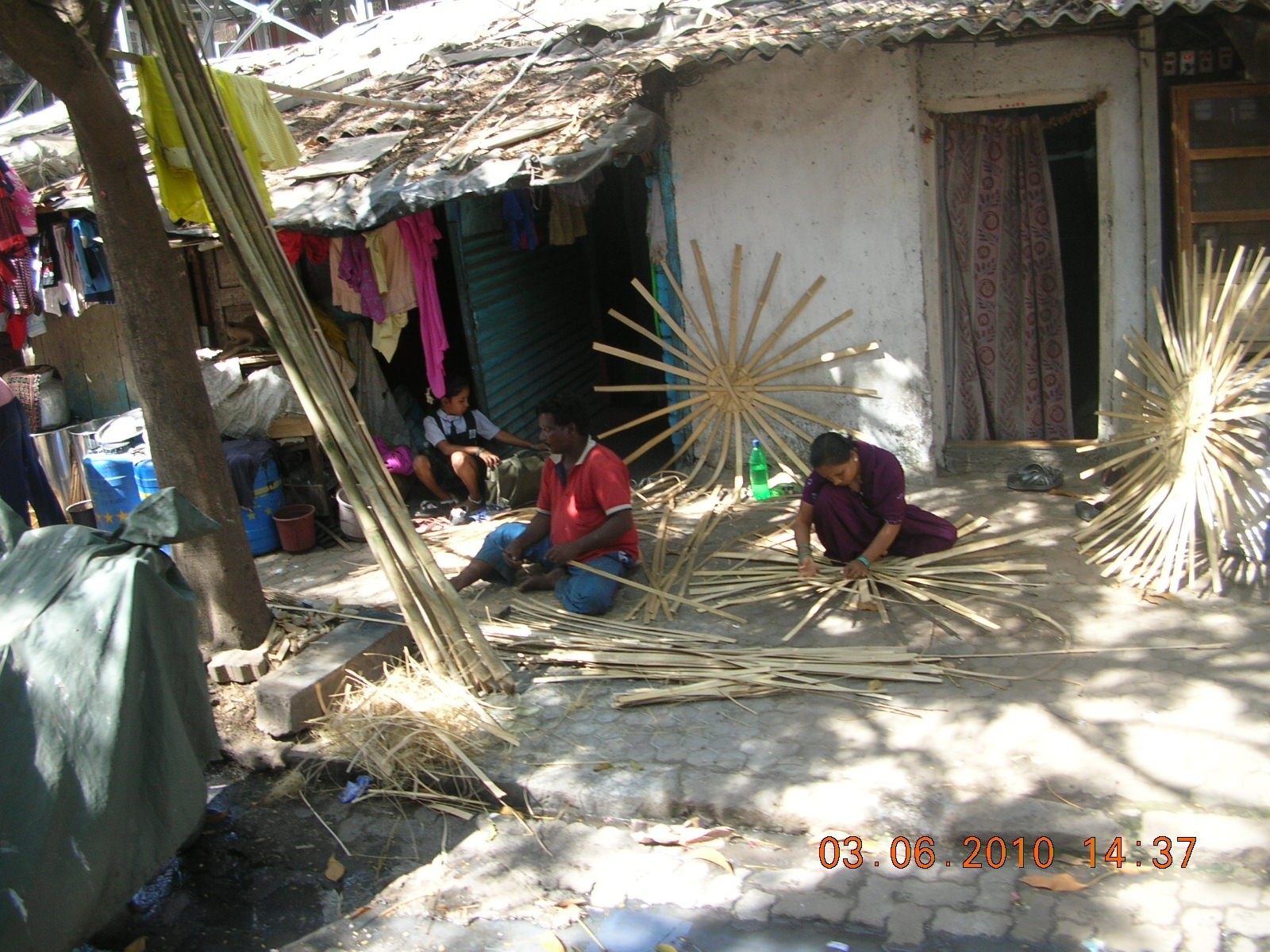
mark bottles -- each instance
[749,439,770,500]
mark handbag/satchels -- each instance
[484,448,553,515]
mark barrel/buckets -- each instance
[272,503,315,554]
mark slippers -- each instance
[1007,462,1064,492]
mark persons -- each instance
[411,374,551,512]
[0,375,68,529]
[794,432,957,579]
[449,393,639,614]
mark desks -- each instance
[274,435,337,502]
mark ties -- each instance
[443,418,457,437]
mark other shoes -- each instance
[414,499,446,518]
[449,504,471,524]
[1075,500,1106,523]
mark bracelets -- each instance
[531,443,535,449]
[476,448,484,456]
[857,556,870,568]
[798,544,813,559]
